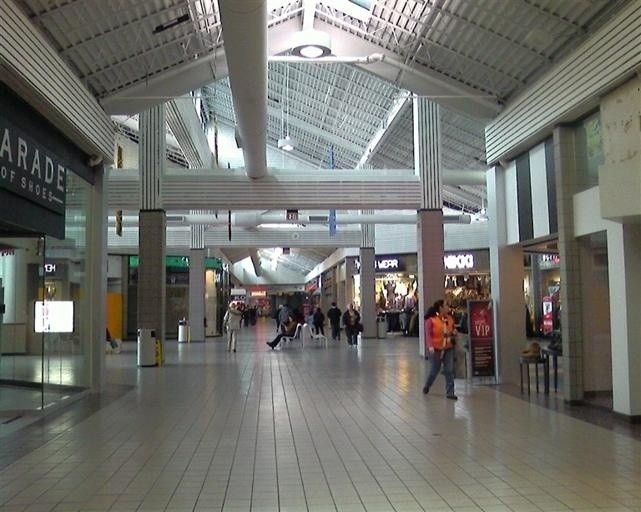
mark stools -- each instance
[520,356,546,396]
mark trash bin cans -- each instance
[377,318,387,339]
[178,320,188,343]
[137,328,157,367]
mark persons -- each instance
[327,302,341,340]
[222,302,257,352]
[423,300,458,400]
[266,302,325,350]
[343,303,360,348]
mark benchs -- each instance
[281,323,328,353]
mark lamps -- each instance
[278,49,298,151]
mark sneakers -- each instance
[447,395,457,400]
[267,342,274,349]
[423,387,429,394]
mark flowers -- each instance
[546,332,562,344]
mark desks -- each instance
[542,348,562,397]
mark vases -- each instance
[548,343,559,350]
